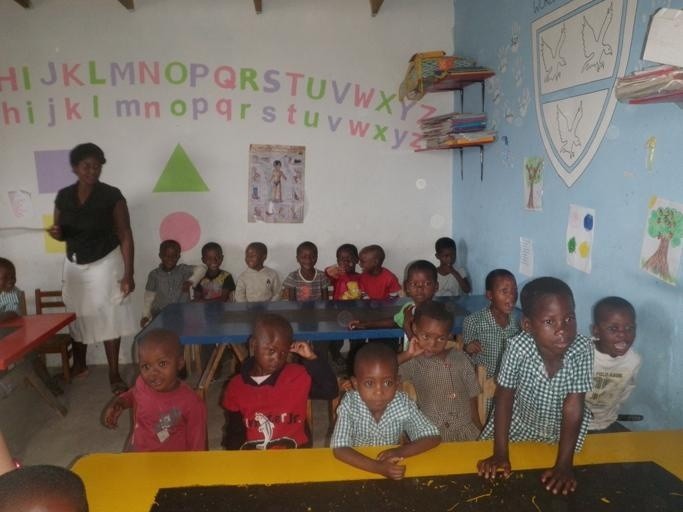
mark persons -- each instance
[1,465,89,512]
[46,144,136,395]
[0,258,27,317]
[267,159,287,204]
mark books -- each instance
[411,112,498,148]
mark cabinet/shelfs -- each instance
[413,69,496,182]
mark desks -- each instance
[1,313,74,471]
[62,429,683,512]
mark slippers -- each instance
[108,372,129,396]
[52,365,90,384]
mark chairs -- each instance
[123,284,527,454]
[17,291,27,316]
[27,288,80,383]
